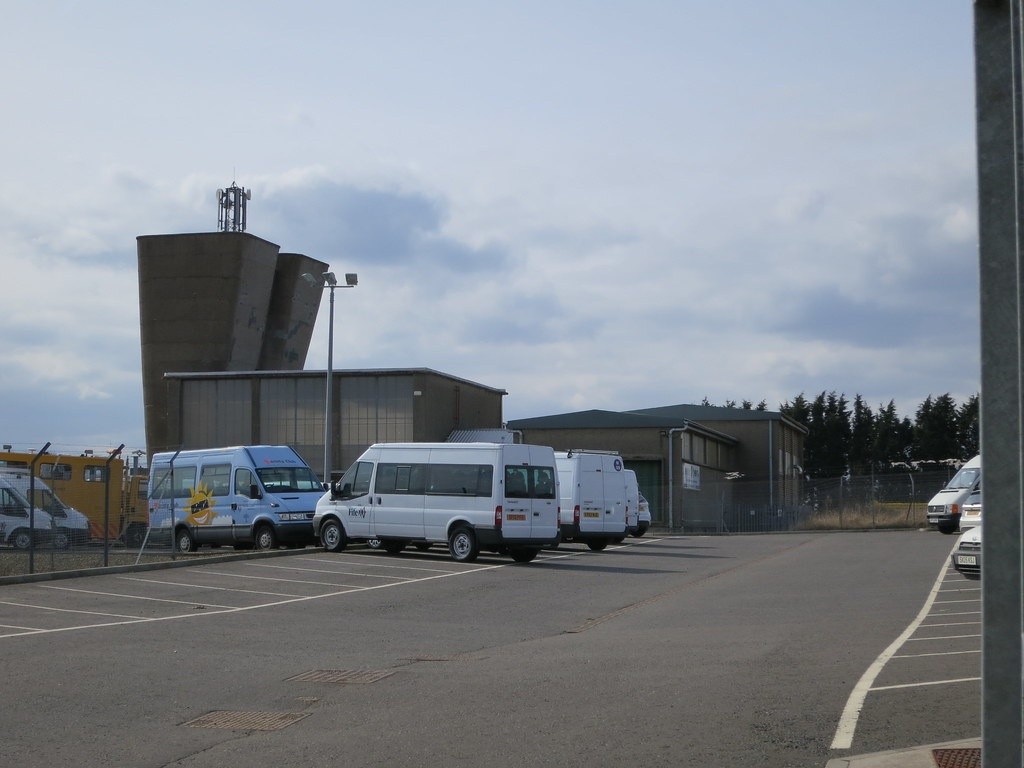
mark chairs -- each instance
[534,472,551,494]
[505,472,525,494]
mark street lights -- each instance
[319,271,358,480]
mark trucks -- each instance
[0,452,151,547]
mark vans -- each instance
[147,444,329,550]
[0,477,58,552]
[504,449,628,552]
[0,470,93,551]
[610,469,640,545]
[313,441,562,563]
[925,454,982,534]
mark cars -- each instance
[959,490,983,533]
[630,491,652,537]
[951,525,982,578]
